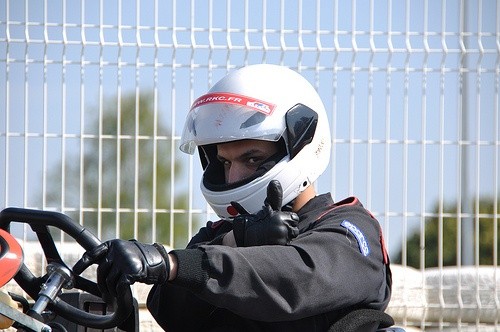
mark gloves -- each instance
[73,238,170,314]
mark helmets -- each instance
[196,63,332,223]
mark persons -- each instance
[73,62,393,332]
